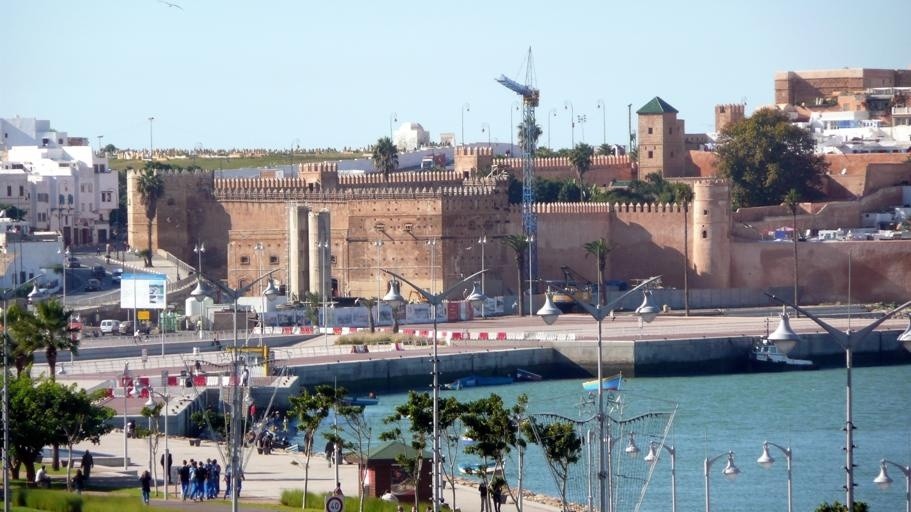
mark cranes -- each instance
[498,37,540,292]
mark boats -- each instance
[462,429,478,445]
[749,338,788,368]
[514,367,542,381]
[441,374,513,390]
[582,370,623,391]
[457,460,506,475]
[343,395,378,406]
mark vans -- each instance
[101,320,121,335]
[119,321,146,334]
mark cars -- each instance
[91,265,106,277]
[68,257,80,268]
[85,280,101,290]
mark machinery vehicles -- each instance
[535,265,593,313]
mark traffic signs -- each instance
[325,495,345,512]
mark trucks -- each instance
[112,268,123,283]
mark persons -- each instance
[325,439,336,467]
[127,420,132,431]
[479,479,489,511]
[194,360,202,376]
[179,456,246,502]
[161,448,173,483]
[195,317,202,333]
[134,329,143,343]
[491,485,503,511]
[130,420,141,438]
[426,505,435,511]
[411,505,417,511]
[212,338,221,345]
[243,410,290,454]
[332,481,345,497]
[382,488,401,501]
[397,505,405,511]
[140,471,153,506]
[81,450,94,478]
[72,469,83,496]
[35,464,52,488]
[145,326,152,340]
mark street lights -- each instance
[97,135,103,148]
[122,362,131,469]
[191,267,280,511]
[538,244,662,512]
[547,110,556,150]
[627,103,634,150]
[317,240,329,327]
[427,237,439,318]
[194,238,206,274]
[379,267,488,512]
[478,234,487,318]
[462,103,469,146]
[762,292,911,511]
[482,122,490,147]
[148,117,154,158]
[254,242,264,297]
[598,101,606,145]
[510,101,519,158]
[565,103,574,151]
[609,432,640,510]
[57,247,70,306]
[645,434,677,512]
[874,458,911,512]
[525,234,535,315]
[0,273,49,512]
[390,112,399,144]
[373,239,384,325]
[107,186,125,267]
[146,389,168,500]
[129,380,154,478]
[758,439,792,511]
[290,142,301,190]
[705,452,738,511]
